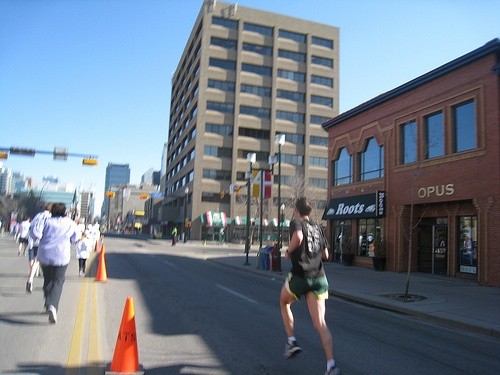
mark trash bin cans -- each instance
[256,245,281,271]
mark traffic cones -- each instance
[105,296,146,375]
[95,240,99,253]
[100,242,106,262]
[96,253,109,282]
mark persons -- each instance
[16,202,100,324]
[464,236,471,253]
[279,197,340,375]
[171,226,177,246]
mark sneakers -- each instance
[324,366,341,375]
[17,252,27,257]
[281,342,302,359]
[78,273,86,276]
[45,304,57,325]
[25,281,33,293]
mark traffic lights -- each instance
[106,191,115,198]
[139,194,148,199]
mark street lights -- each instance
[274,131,286,271]
[246,152,279,250]
[183,186,189,243]
[244,171,253,265]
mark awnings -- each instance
[200,211,291,228]
